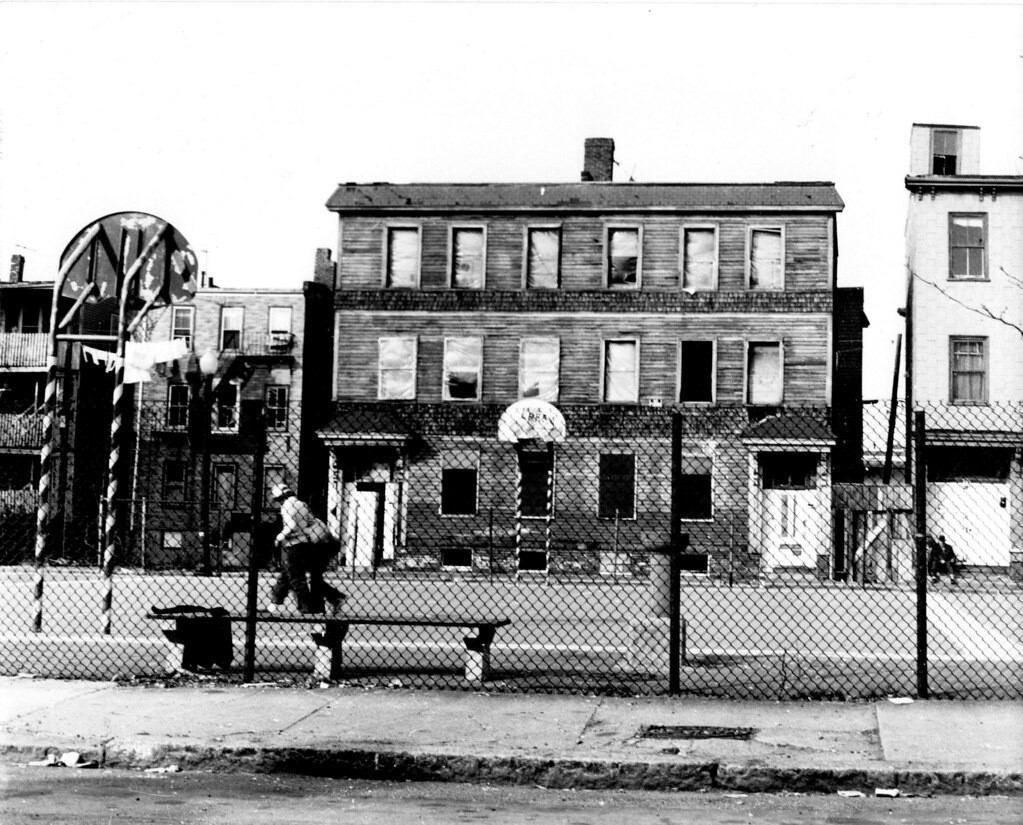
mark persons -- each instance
[929,535,957,584]
[266,484,349,614]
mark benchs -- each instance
[148,614,509,681]
[958,564,1009,573]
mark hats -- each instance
[271,484,293,503]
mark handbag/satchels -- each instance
[314,533,341,562]
